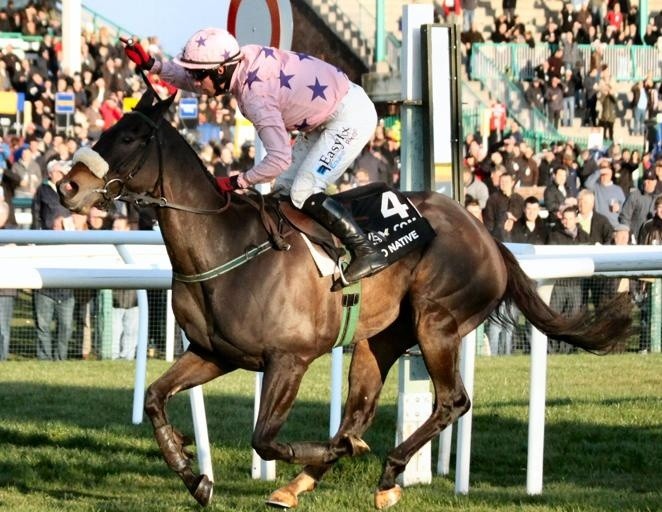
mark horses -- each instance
[49,85,644,512]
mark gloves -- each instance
[118,36,155,70]
[213,174,243,192]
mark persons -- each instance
[0,3,257,362]
[433,1,661,78]
[118,25,390,292]
[324,118,401,198]
[525,78,661,143]
[461,124,661,360]
[491,98,509,140]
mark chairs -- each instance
[556,76,650,146]
[355,0,660,46]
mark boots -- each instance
[312,197,390,292]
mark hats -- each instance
[642,169,656,180]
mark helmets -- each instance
[172,27,244,71]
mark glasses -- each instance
[189,69,210,80]
